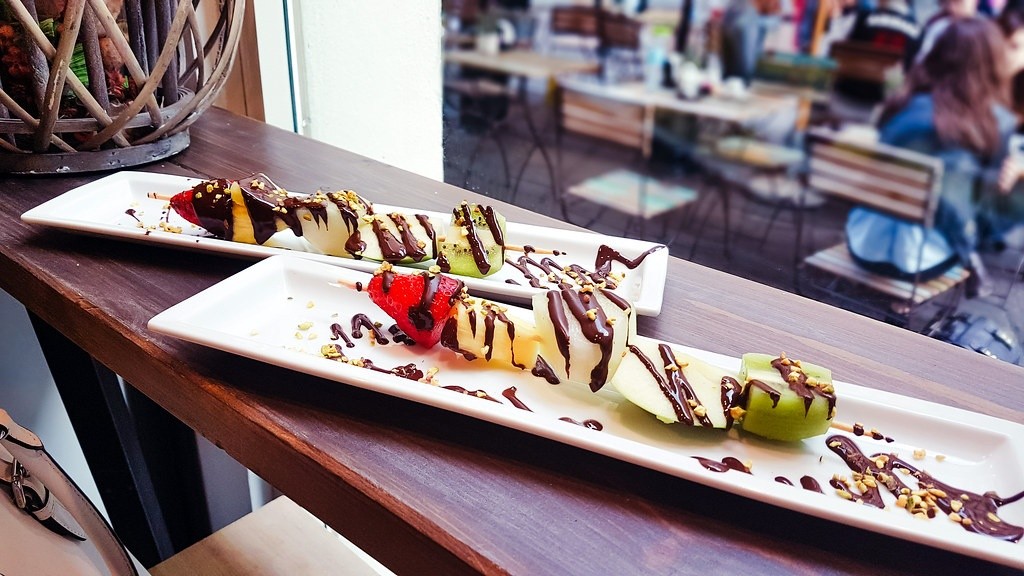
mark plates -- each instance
[21,170,670,318]
[146,255,1024,569]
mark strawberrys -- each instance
[170,179,233,230]
[367,272,464,349]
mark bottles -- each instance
[643,25,668,89]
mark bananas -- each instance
[455,304,540,370]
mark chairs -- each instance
[544,81,780,263]
[552,83,700,253]
[792,123,970,342]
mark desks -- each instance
[0,104,1024,576]
[448,49,598,205]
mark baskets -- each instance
[0,0,246,173]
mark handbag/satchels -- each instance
[0,409,153,576]
[929,313,1021,364]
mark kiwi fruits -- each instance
[734,352,835,441]
[435,203,507,277]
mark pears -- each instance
[531,288,639,386]
[291,191,369,258]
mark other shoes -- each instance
[967,254,994,299]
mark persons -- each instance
[840,15,1024,340]
[700,1,1024,127]
[441,0,530,141]
